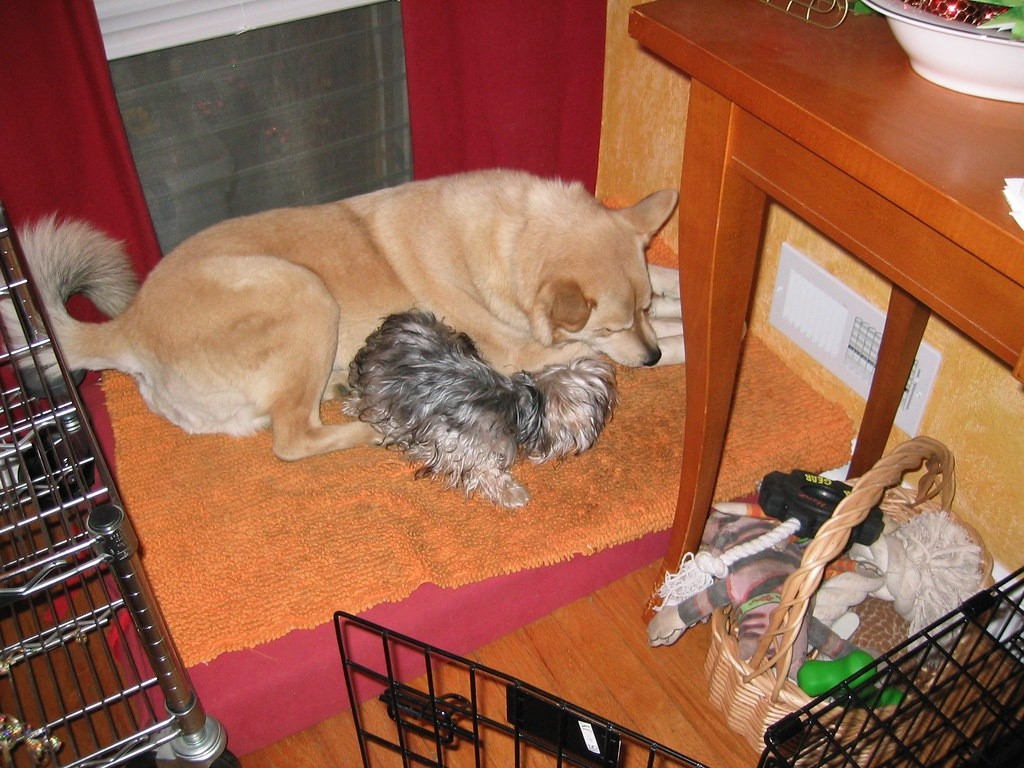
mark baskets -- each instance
[702,434,994,768]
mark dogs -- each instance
[1,168,679,461]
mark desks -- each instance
[624,0,1024,629]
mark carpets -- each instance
[93,193,853,658]
[73,371,759,768]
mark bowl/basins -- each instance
[859,0,1024,104]
